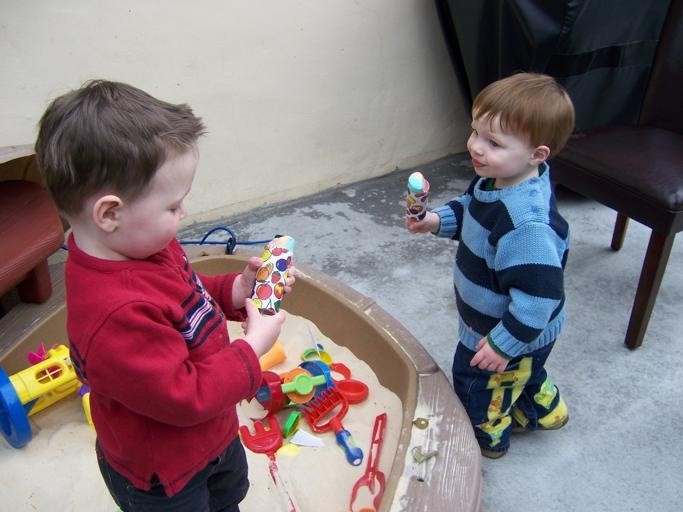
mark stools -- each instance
[0,178,65,321]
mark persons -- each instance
[406,72,576,460]
[33,80,296,512]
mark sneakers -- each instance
[476,391,569,459]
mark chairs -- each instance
[492,1,682,352]
